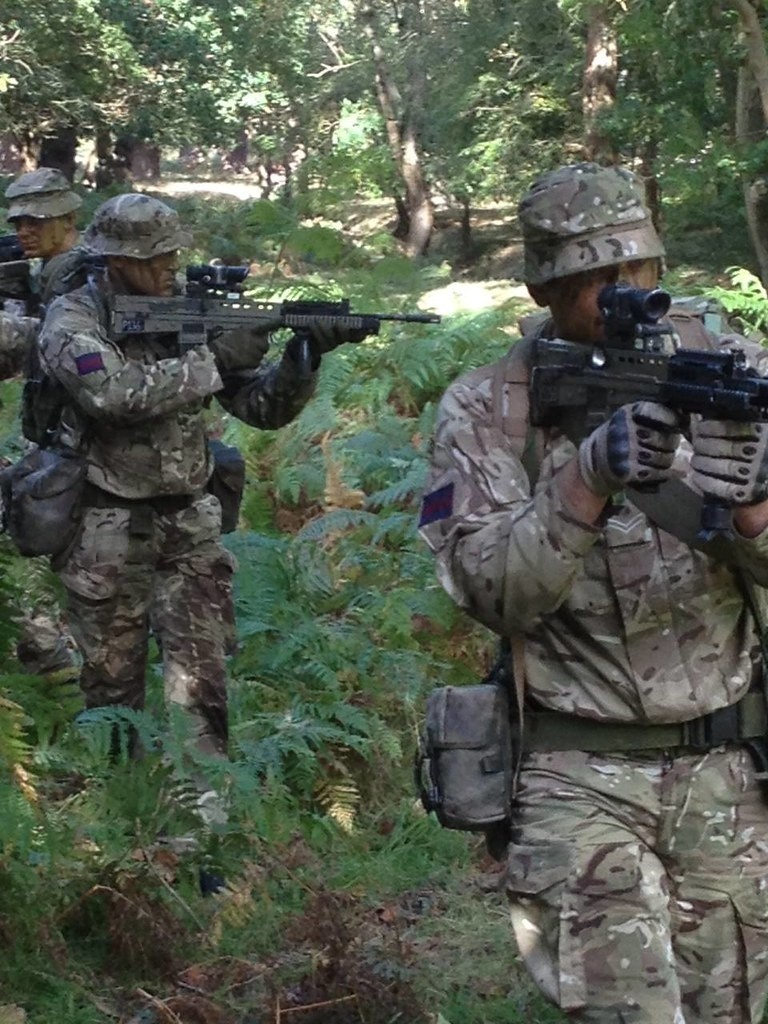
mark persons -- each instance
[0,168,86,450]
[415,164,768,1024]
[22,198,362,770]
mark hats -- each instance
[76,193,206,263]
[522,162,686,285]
[4,166,83,222]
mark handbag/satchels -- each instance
[210,439,249,533]
[3,446,94,560]
[419,681,519,831]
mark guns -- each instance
[108,258,442,383]
[527,283,768,429]
[0,234,24,264]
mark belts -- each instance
[77,476,205,535]
[503,691,767,758]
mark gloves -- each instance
[575,399,680,498]
[689,415,768,499]
[207,323,274,372]
[300,312,370,354]
